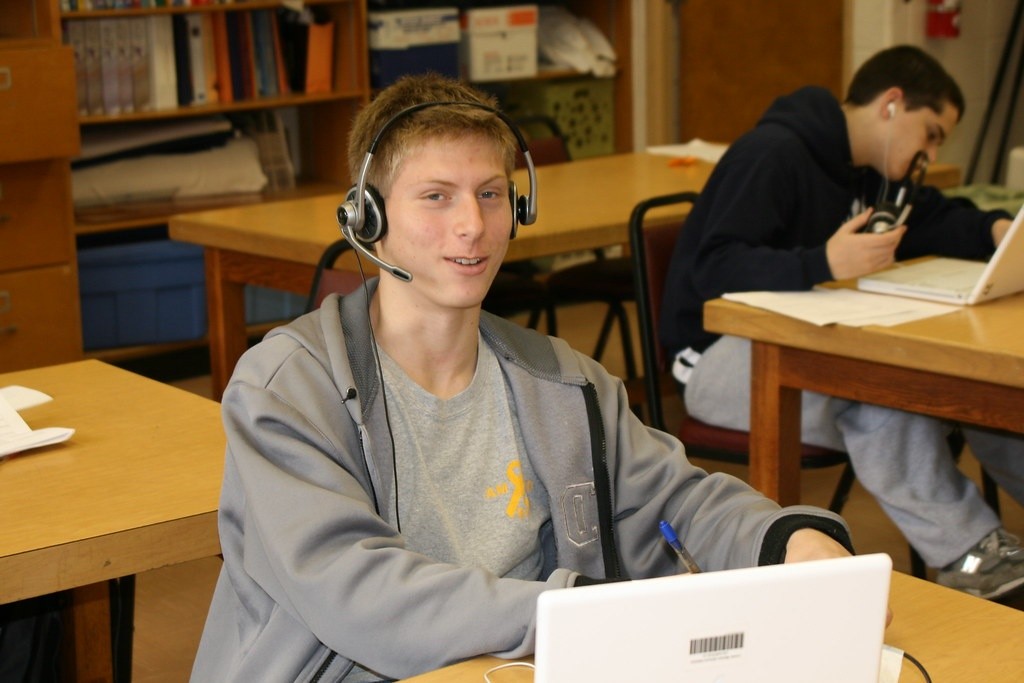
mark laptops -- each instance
[534,551,892,683]
[858,203,1024,305]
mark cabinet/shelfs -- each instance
[0,1,82,373]
[56,0,635,360]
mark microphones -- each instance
[341,226,412,282]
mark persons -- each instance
[189,73,857,683]
[658,40,1023,601]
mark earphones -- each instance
[887,101,896,119]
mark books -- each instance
[62,1,283,117]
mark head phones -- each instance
[335,101,538,244]
[865,151,929,235]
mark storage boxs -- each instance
[506,74,618,157]
[364,5,462,87]
[460,5,541,83]
[76,239,307,353]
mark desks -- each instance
[394,568,1024,683]
[703,253,1023,513]
[0,359,223,683]
[168,139,967,408]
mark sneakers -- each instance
[932,526,1024,599]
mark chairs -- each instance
[631,190,1004,581]
[512,111,646,428]
